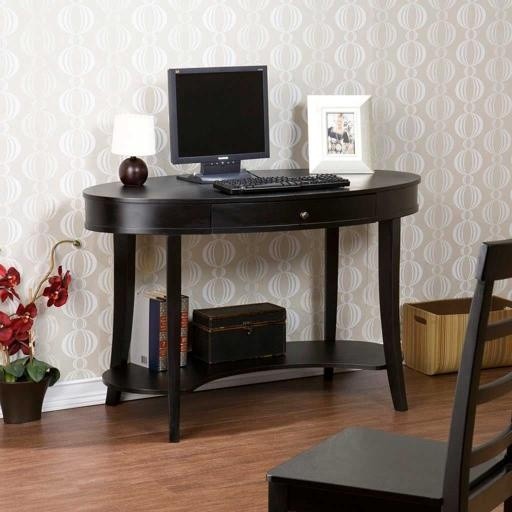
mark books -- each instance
[127,292,188,372]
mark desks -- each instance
[82,166,422,444]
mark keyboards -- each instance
[212,173,352,196]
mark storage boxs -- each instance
[191,302,287,366]
[401,293,512,377]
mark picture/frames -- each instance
[304,93,377,177]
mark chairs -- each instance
[262,237,512,512]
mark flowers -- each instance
[0,238,83,387]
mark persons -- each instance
[327,114,349,154]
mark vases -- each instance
[0,371,52,424]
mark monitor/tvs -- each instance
[167,65,271,184]
